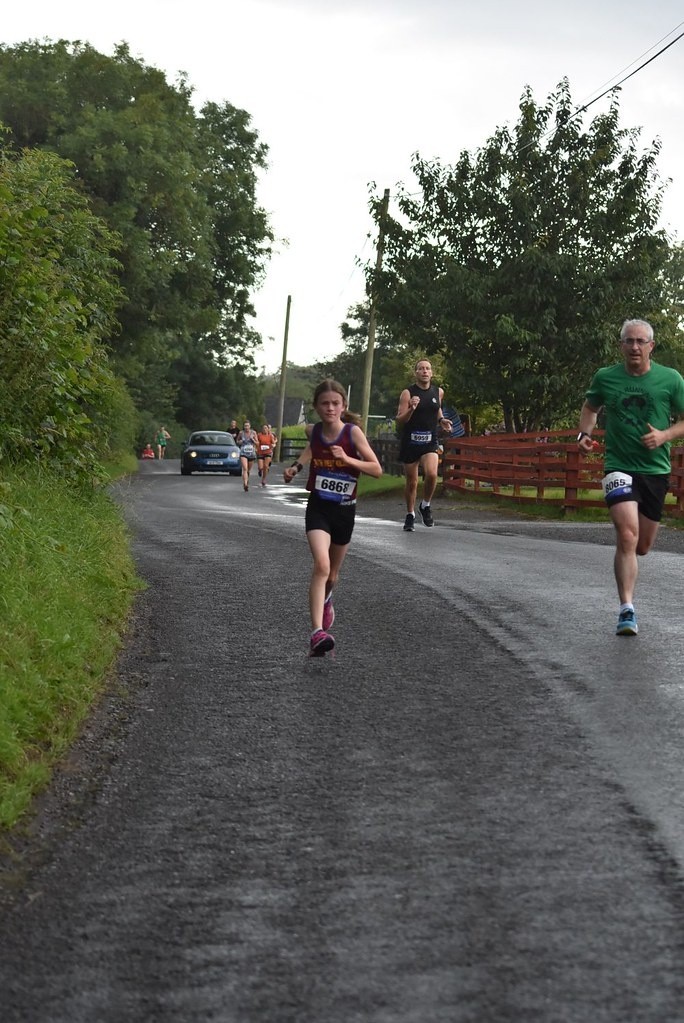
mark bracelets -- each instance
[439,417,443,425]
[291,461,303,472]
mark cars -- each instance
[180,430,242,476]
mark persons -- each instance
[226,419,242,448]
[256,424,277,487]
[237,420,259,492]
[283,380,382,657]
[142,444,154,460]
[576,320,684,636]
[440,406,465,484]
[396,359,453,532]
[155,426,171,460]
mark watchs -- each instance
[577,432,590,442]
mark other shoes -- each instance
[262,480,265,485]
[244,486,248,492]
[259,471,262,477]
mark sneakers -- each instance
[308,630,335,658]
[615,608,639,636]
[417,502,435,528]
[322,590,335,632]
[403,514,416,532]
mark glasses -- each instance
[621,338,650,345]
